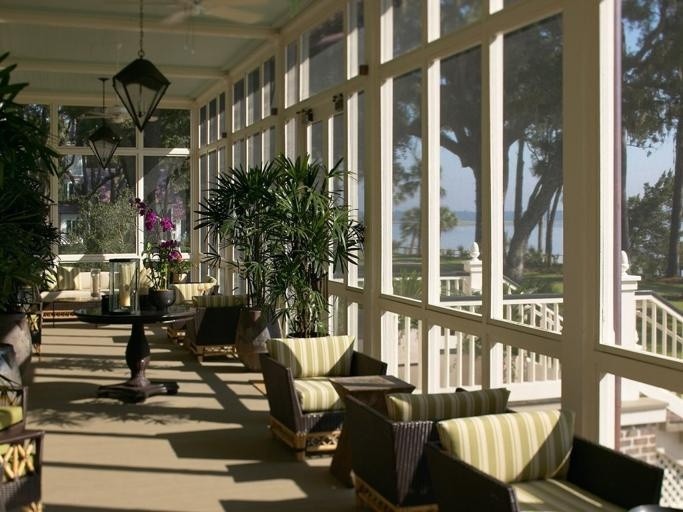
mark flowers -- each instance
[129,196,183,291]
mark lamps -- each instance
[112,1,171,133]
[88,77,124,171]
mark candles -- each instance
[119,265,131,309]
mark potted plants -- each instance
[193,153,368,374]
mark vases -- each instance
[148,288,176,315]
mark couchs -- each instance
[34,272,118,328]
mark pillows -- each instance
[43,269,56,292]
[57,267,81,290]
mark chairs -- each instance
[428,407,664,510]
[257,334,388,464]
[342,387,519,511]
[0,386,44,512]
[169,276,264,349]
[8,290,43,355]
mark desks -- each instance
[70,307,197,403]
[329,375,416,489]
